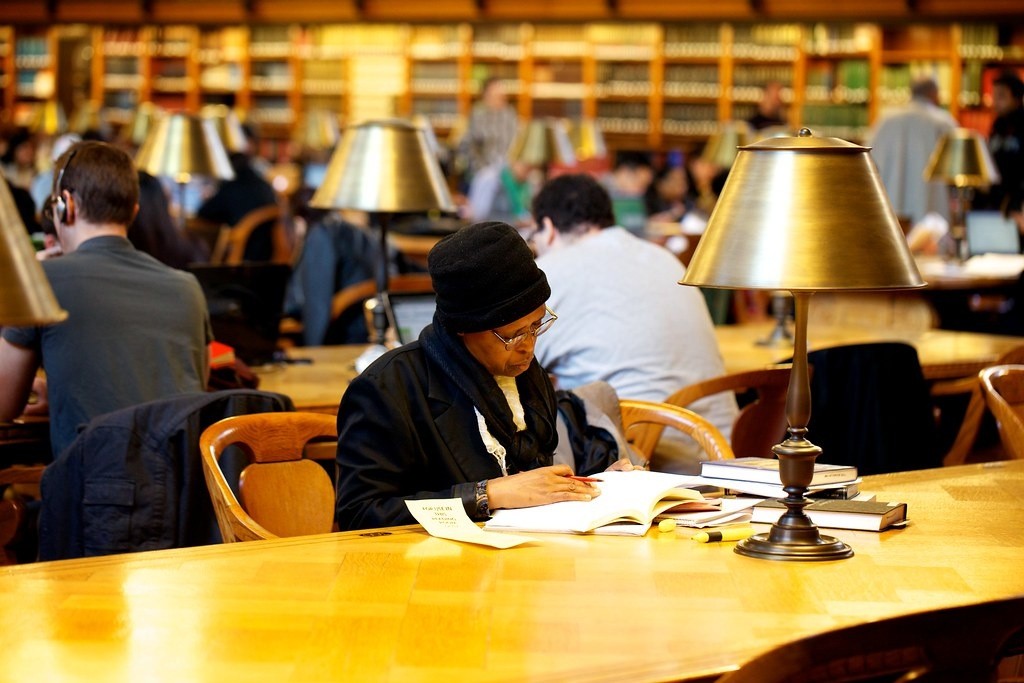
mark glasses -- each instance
[45,199,59,220]
[492,304,558,352]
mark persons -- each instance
[970,74,1024,230]
[129,126,330,340]
[336,222,646,531]
[871,75,962,233]
[530,172,740,473]
[748,81,789,133]
[646,153,728,224]
[0,142,214,457]
[458,77,532,218]
[0,128,106,221]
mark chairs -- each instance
[0,200,1023,545]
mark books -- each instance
[0,23,1024,158]
[485,457,907,537]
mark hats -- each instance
[426,220,552,334]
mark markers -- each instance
[691,527,753,543]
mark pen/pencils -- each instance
[518,470,603,482]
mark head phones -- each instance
[56,147,80,223]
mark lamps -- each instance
[303,117,455,345]
[677,128,934,561]
[120,99,252,226]
[0,171,72,327]
[920,131,997,256]
[515,118,575,195]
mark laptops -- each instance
[968,209,1020,257]
[189,262,292,367]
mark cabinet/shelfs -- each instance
[0,17,1024,166]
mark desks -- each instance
[917,260,1023,328]
[0,314,1022,443]
[0,462,1024,683]
